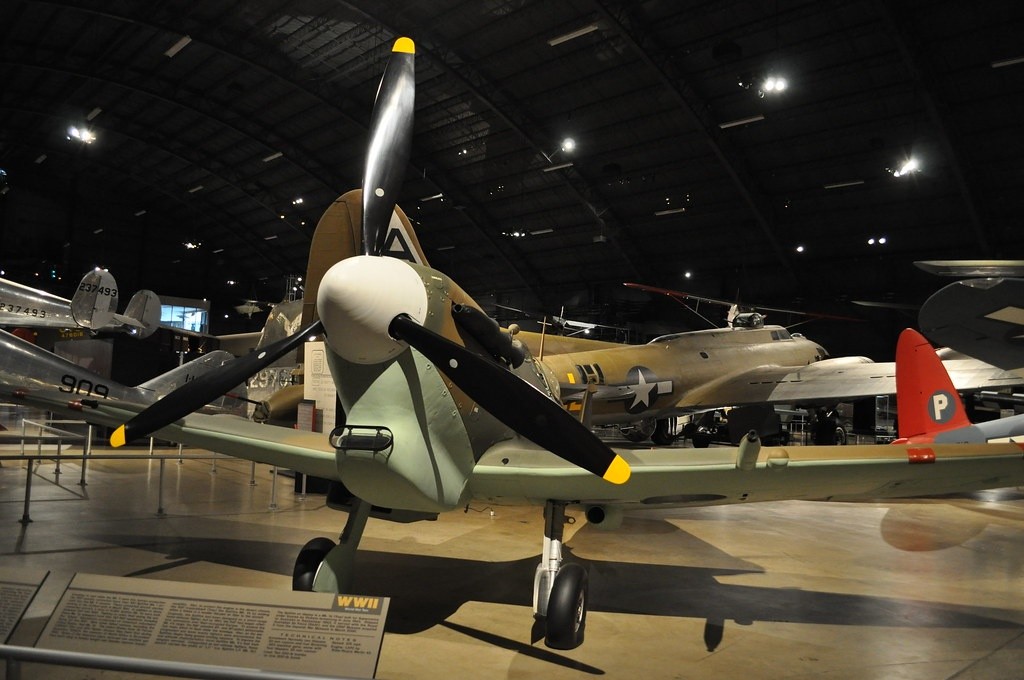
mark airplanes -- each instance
[0,34,1024,653]
